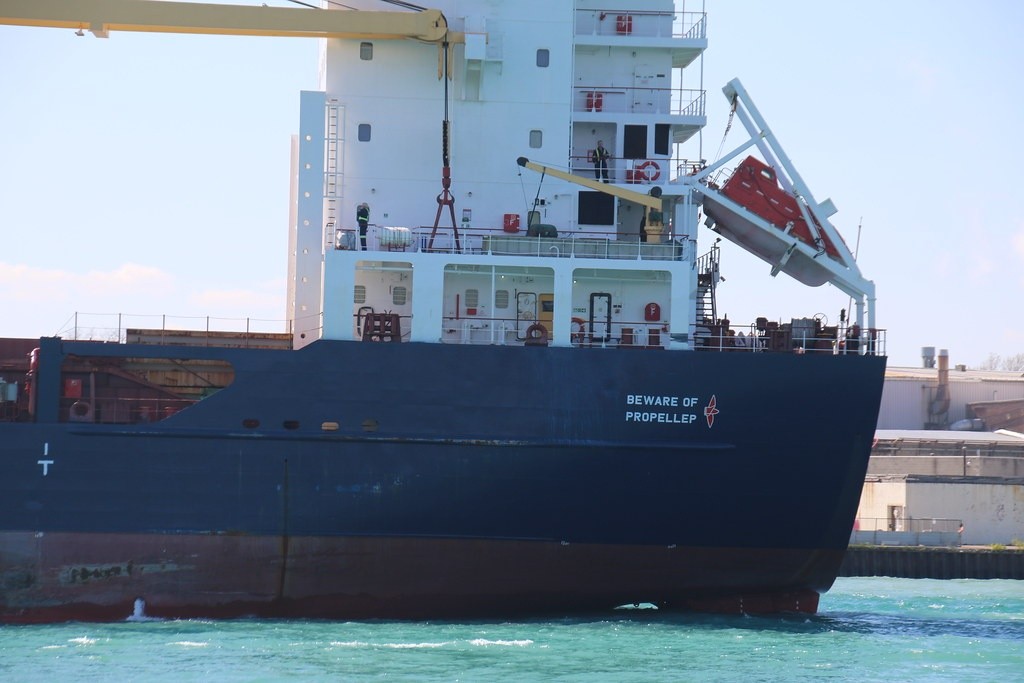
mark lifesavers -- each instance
[641,161,660,181]
[526,324,548,341]
[70,401,93,421]
[570,317,585,338]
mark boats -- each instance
[0,0,895,627]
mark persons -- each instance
[592,140,610,183]
[356,202,371,251]
